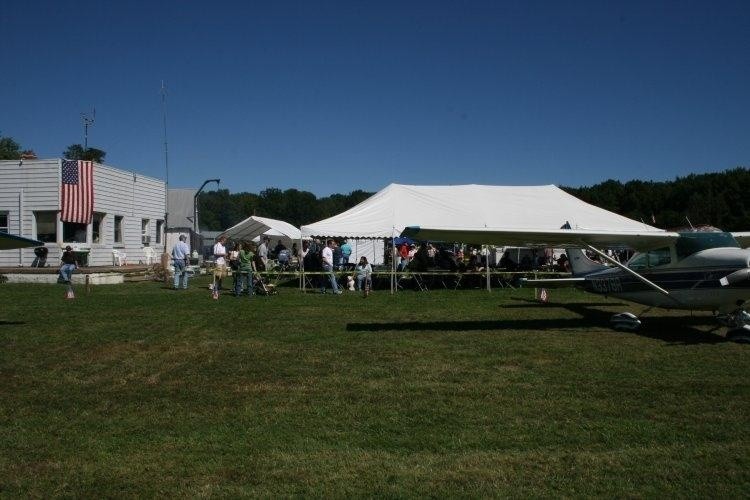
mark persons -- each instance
[291,238,372,294]
[557,253,571,272]
[172,235,190,291]
[60,245,79,282]
[213,236,287,296]
[498,251,531,269]
[30,243,47,267]
[394,241,484,272]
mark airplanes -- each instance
[0,231,46,253]
[401,217,749,342]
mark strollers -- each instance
[250,271,280,297]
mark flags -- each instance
[60,159,94,224]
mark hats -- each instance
[263,236,272,240]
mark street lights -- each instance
[191,178,222,257]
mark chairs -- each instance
[112,250,127,267]
[143,247,157,263]
